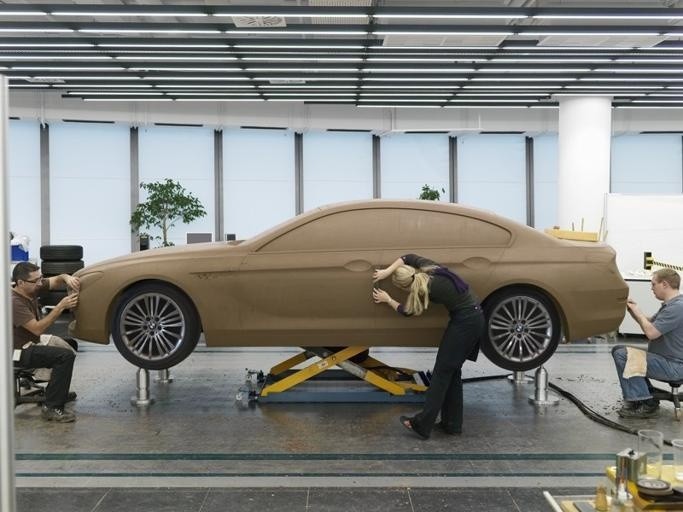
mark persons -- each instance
[12,261,80,423]
[610,268,683,420]
[371,253,488,442]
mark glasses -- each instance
[25,276,43,284]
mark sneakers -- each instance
[433,423,462,436]
[65,392,76,401]
[619,400,659,418]
[41,404,75,422]
[400,416,428,440]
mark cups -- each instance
[638,430,663,478]
[672,440,683,481]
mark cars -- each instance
[65,200,630,372]
